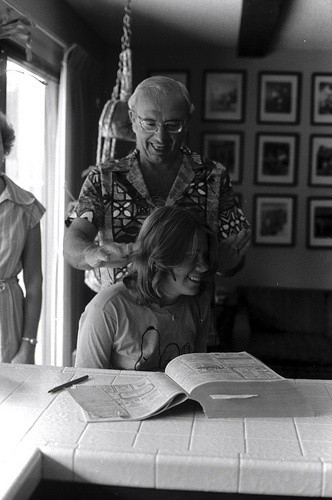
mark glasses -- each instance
[132,109,185,133]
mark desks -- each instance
[0,362,332,500]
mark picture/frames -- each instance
[200,69,247,125]
[308,133,332,188]
[256,69,302,125]
[201,131,244,184]
[306,195,332,250]
[311,71,332,126]
[251,194,297,247]
[253,131,300,186]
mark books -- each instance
[70,350,314,423]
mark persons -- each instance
[0,100,47,364]
[76,206,217,372]
[61,76,251,362]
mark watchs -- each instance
[21,337,37,344]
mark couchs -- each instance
[229,286,332,365]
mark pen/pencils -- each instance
[48,375,89,393]
